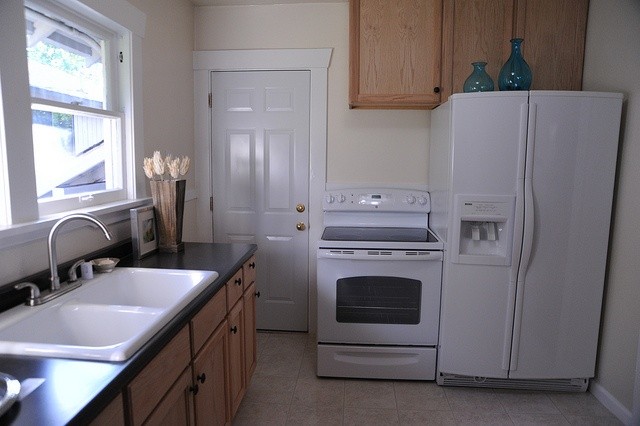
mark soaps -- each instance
[99,258,115,269]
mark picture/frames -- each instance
[130,204,159,259]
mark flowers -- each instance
[143,149,193,180]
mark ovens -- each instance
[317,226,443,380]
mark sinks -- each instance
[15,211,117,310]
[0,302,171,363]
[67,264,219,311]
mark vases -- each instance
[463,61,494,91]
[498,39,531,89]
[149,180,186,253]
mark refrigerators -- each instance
[429,90,623,393]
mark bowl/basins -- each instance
[90,258,120,273]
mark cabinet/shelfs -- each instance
[243,253,259,394]
[128,285,229,426]
[348,0,441,110]
[225,267,245,424]
[442,0,590,105]
